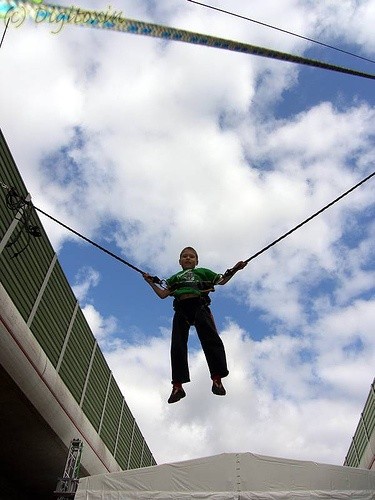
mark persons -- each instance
[141,246,248,403]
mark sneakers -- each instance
[168,386,186,404]
[212,379,225,395]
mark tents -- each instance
[75,453,375,500]
